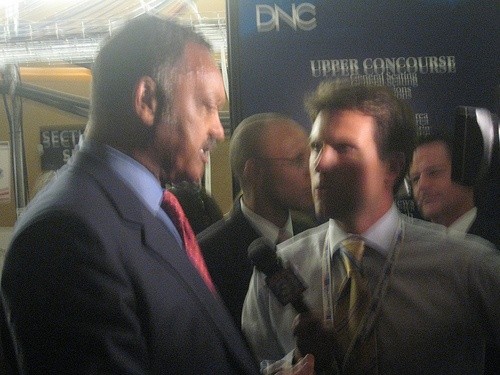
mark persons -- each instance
[185,81,498,375]
[0,12,258,375]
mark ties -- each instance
[160,189,217,302]
[332,239,378,375]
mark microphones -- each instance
[249,239,309,314]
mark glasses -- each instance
[264,154,305,164]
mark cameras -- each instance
[450,105,500,187]
